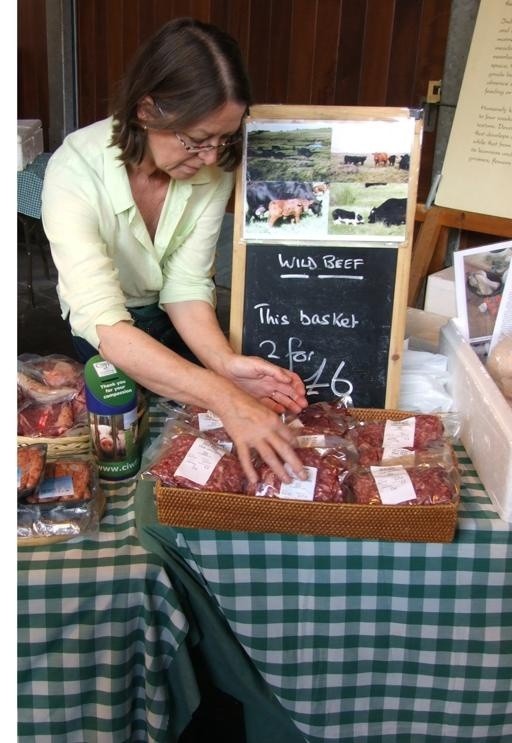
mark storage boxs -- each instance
[438,317,512,523]
[424,266,460,315]
[19,118,45,170]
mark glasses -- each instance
[153,99,244,153]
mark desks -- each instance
[18,152,61,307]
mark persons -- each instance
[39,14,308,484]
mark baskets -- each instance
[17,387,148,464]
